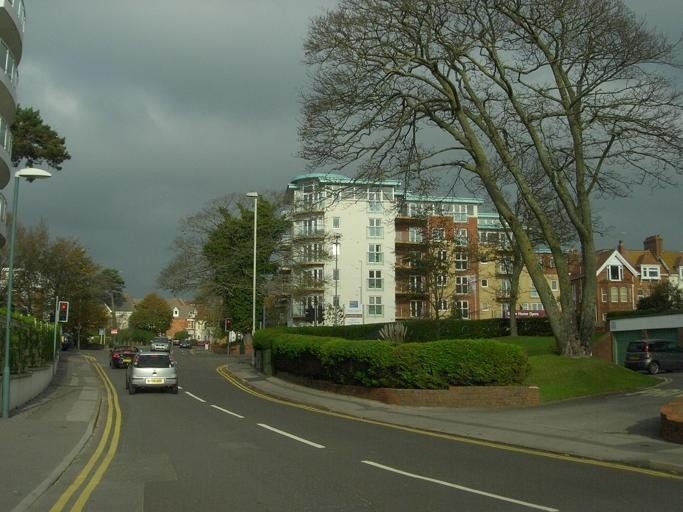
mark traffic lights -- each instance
[225,317,232,332]
[57,301,68,322]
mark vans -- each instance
[624,338,683,375]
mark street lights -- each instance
[2,167,52,419]
[246,191,258,337]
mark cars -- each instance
[151,335,171,353]
[126,352,177,394]
[109,344,142,369]
[173,337,192,349]
